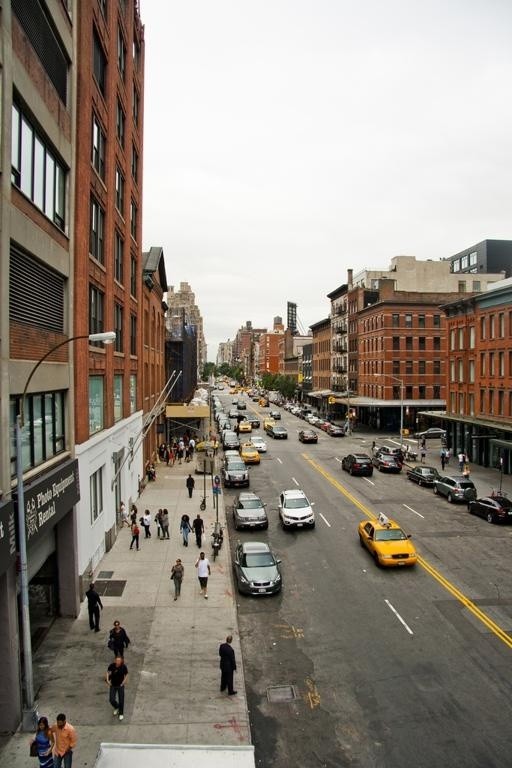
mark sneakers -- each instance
[113,708,119,715]
[200,590,203,594]
[119,715,123,720]
[204,595,207,598]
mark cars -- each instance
[233,492,268,530]
[293,404,331,432]
[211,395,229,422]
[229,409,239,417]
[239,418,252,432]
[469,496,512,523]
[270,410,281,419]
[359,513,417,569]
[299,430,318,442]
[238,402,246,409]
[267,424,287,438]
[415,428,447,439]
[372,453,402,472]
[278,489,315,529]
[233,542,281,594]
[407,466,442,485]
[215,375,266,404]
[239,443,260,464]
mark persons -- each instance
[106,656,128,720]
[171,559,184,600]
[186,474,194,497]
[129,520,140,551]
[219,636,237,695]
[146,461,156,480]
[193,515,204,548]
[32,717,54,768]
[142,510,152,538]
[214,475,221,494]
[154,509,170,540]
[441,448,450,471]
[50,713,77,768]
[419,443,427,464]
[458,451,471,472]
[109,620,130,657]
[129,505,137,525]
[86,583,103,632]
[119,502,130,528]
[180,515,192,547]
[234,425,241,436]
[159,431,195,465]
[196,552,211,599]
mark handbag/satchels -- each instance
[30,734,39,757]
[108,638,114,650]
[170,566,174,579]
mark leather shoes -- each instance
[221,687,227,691]
[229,691,237,695]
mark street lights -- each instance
[16,332,117,729]
[373,374,403,445]
[208,372,217,436]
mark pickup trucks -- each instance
[284,403,294,411]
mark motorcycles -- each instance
[211,530,223,562]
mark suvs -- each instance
[223,432,240,450]
[217,418,231,432]
[222,458,251,486]
[263,418,277,430]
[434,476,477,503]
[342,454,373,475]
[247,417,260,428]
[327,425,345,436]
[249,437,267,453]
[376,446,404,463]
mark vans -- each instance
[221,450,240,463]
